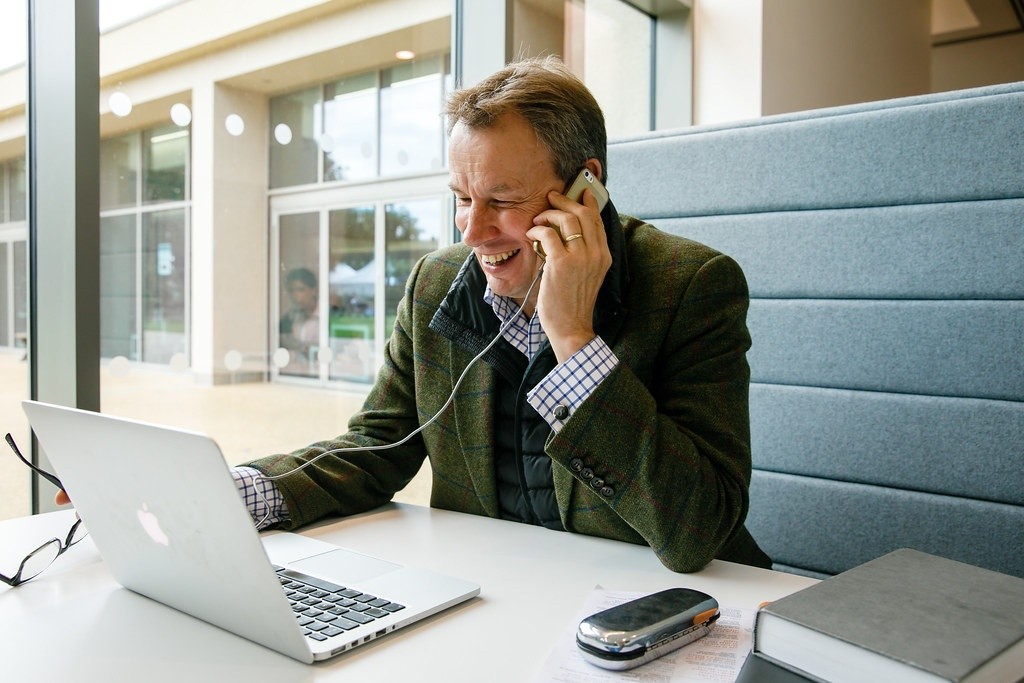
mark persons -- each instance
[55,53,773,576]
[272,266,329,377]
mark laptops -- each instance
[21,400,480,665]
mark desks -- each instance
[0,501,825,683]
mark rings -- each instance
[564,233,584,242]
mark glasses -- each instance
[0,433,89,586]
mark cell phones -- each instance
[533,166,609,262]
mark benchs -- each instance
[605,79,1024,581]
